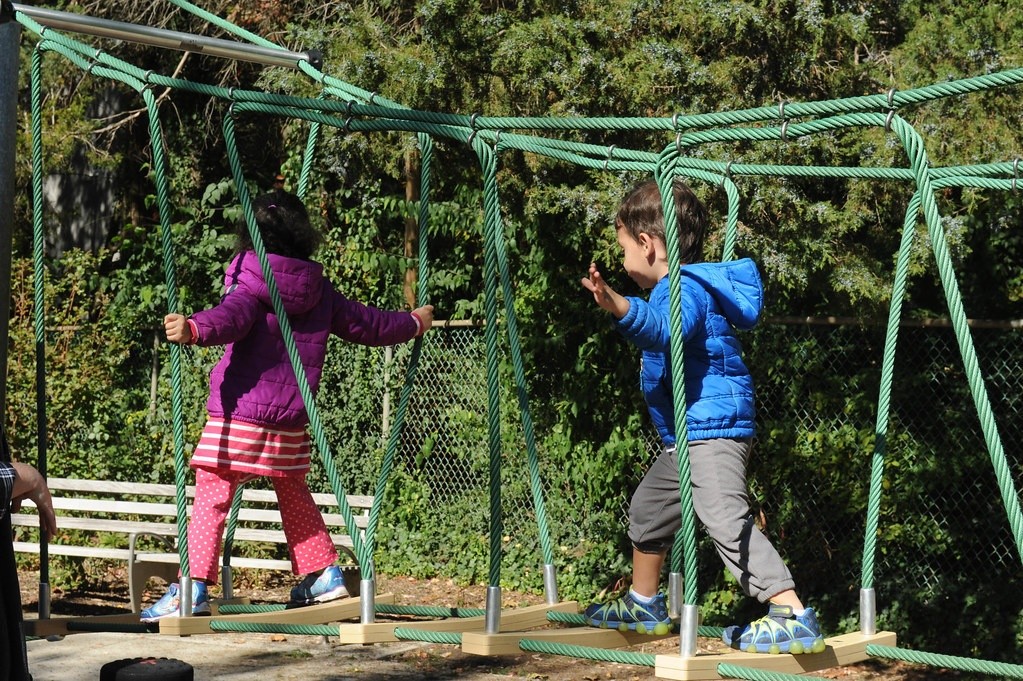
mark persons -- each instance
[581,177,827,654]
[139,191,434,623]
[0,462,58,681]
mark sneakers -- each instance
[583,576,675,636]
[720,600,826,654]
[139,581,211,623]
[289,563,348,603]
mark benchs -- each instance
[9,476,381,614]
